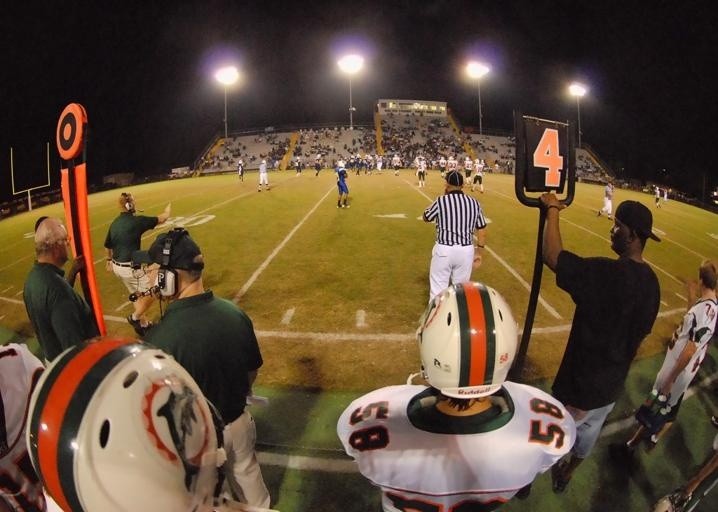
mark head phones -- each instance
[153,227,190,299]
[121,193,133,211]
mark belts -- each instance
[112,262,130,267]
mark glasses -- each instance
[57,236,71,245]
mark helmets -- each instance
[417,153,482,165]
[337,161,345,168]
[347,153,374,160]
[25,336,218,512]
[415,282,519,400]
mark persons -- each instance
[26,334,279,512]
[607,258,718,512]
[336,280,578,512]
[514,193,662,502]
[574,152,613,182]
[598,181,616,221]
[653,187,668,207]
[422,169,487,305]
[129,227,273,510]
[34,216,86,288]
[191,109,513,209]
[0,342,46,511]
[22,217,101,367]
[103,191,172,331]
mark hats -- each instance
[615,200,661,242]
[132,233,204,271]
[445,170,463,186]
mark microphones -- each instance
[129,286,158,302]
[130,208,144,212]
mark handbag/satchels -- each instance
[655,488,692,512]
[635,388,671,434]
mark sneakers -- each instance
[141,321,154,336]
[551,458,572,494]
[337,205,342,208]
[127,314,146,337]
[711,416,718,427]
[342,205,350,208]
[638,428,658,450]
[608,440,634,454]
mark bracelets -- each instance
[548,205,559,211]
[477,244,485,248]
